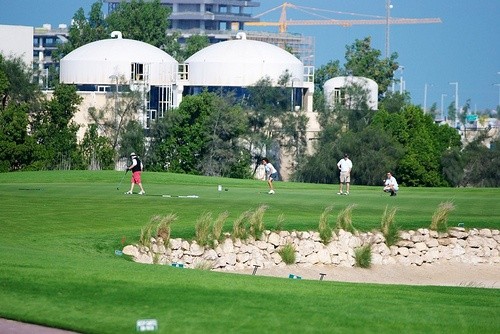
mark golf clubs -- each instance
[117,169,129,190]
[344,177,346,195]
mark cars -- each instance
[434,116,479,130]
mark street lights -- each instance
[450,82,459,127]
[440,94,447,120]
[385,0,394,58]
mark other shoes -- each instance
[268,190,275,194]
[124,191,132,194]
[344,192,349,195]
[340,191,343,194]
[138,191,145,194]
[391,191,396,195]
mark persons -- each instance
[336,153,353,196]
[263,158,278,194]
[126,153,145,195]
[383,171,399,196]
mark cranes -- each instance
[231,2,441,47]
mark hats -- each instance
[130,153,137,156]
[344,154,348,157]
